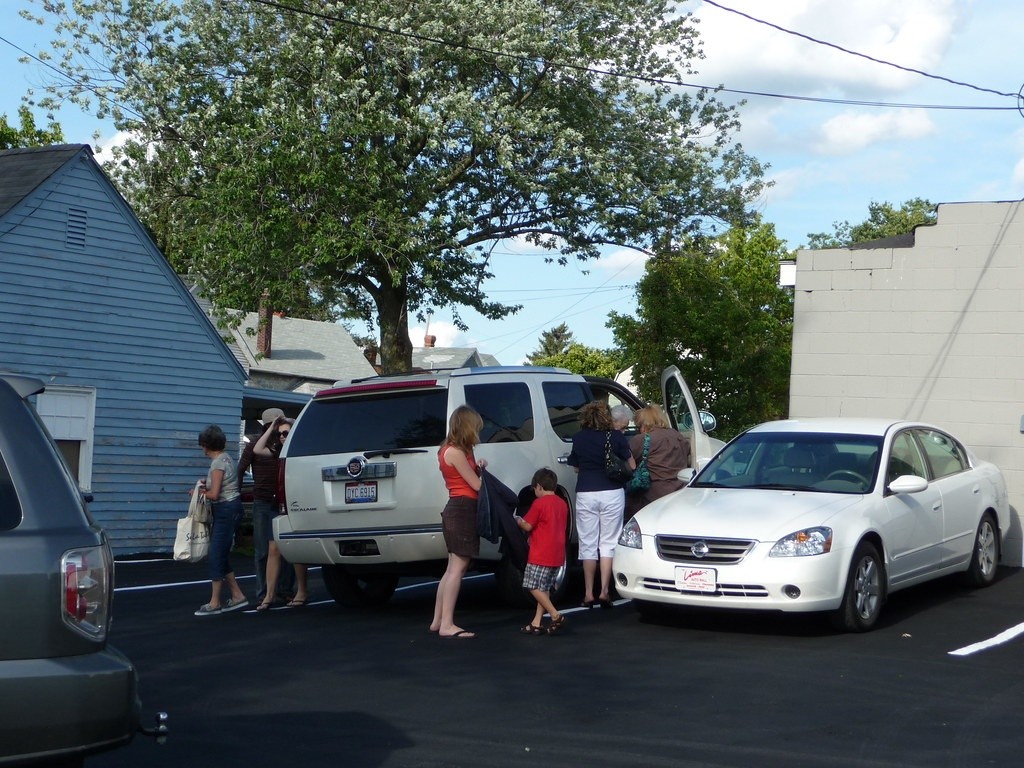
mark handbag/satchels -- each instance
[606,430,633,481]
[173,483,212,563]
[193,493,212,524]
[626,433,651,493]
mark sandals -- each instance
[546,615,567,635]
[520,623,544,635]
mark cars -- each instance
[613,416,1011,636]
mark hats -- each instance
[262,408,285,423]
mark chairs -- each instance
[769,446,901,490]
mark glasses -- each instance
[277,431,288,438]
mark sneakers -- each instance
[194,604,223,615]
[221,597,248,611]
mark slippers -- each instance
[287,599,311,607]
[256,599,277,611]
[439,630,479,639]
[429,630,439,634]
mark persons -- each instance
[568,402,636,608]
[629,405,690,513]
[512,468,568,635]
[188,425,250,616]
[428,405,488,635]
[607,405,632,436]
[237,408,308,611]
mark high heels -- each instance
[581,600,594,608]
[599,599,614,610]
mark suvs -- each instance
[271,364,736,610]
[0,370,166,768]
